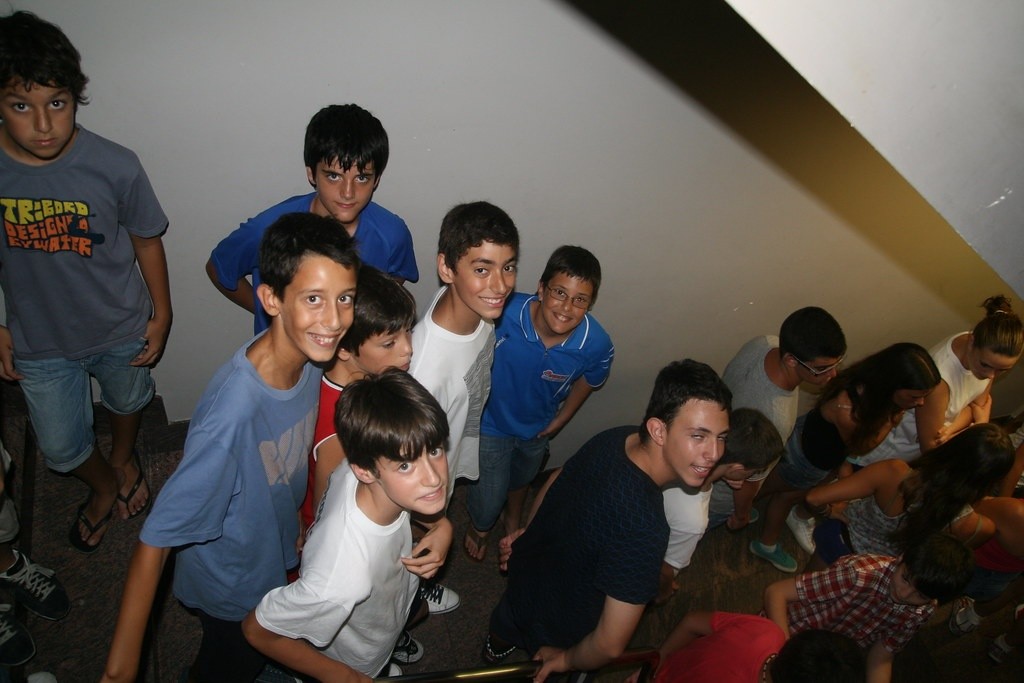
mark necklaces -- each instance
[763,655,778,683]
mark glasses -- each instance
[793,354,848,378]
[546,284,591,309]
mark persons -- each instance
[486,296,1023,683]
[466,245,615,557]
[99,214,357,683]
[206,104,419,337]
[301,273,461,616]
[0,445,71,666]
[1,10,175,550]
[240,365,452,683]
[408,200,521,488]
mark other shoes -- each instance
[392,629,425,663]
[255,664,303,683]
[483,634,519,665]
[376,662,402,677]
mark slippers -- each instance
[69,494,118,554]
[499,562,509,577]
[462,520,490,561]
[117,449,152,524]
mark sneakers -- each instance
[0,549,72,622]
[416,577,461,615]
[984,633,1008,664]
[0,601,36,667]
[748,505,759,523]
[749,538,797,574]
[785,504,817,555]
[948,596,979,637]
